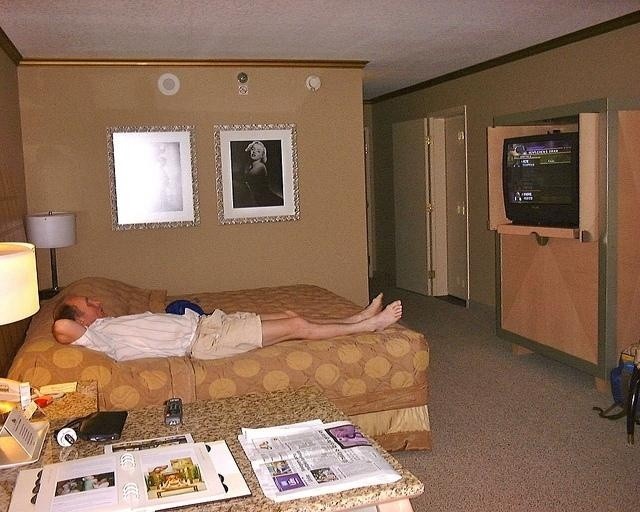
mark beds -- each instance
[5,285,431,453]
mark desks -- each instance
[0,381,424,512]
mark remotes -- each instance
[165,397,182,426]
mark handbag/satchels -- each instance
[610,362,640,425]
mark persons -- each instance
[56,473,114,497]
[160,474,193,491]
[509,142,529,168]
[232,140,285,206]
[50,291,403,363]
[152,466,161,490]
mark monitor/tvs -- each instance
[503,132,579,225]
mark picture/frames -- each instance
[213,123,300,225]
[106,125,200,231]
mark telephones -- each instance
[0,378,34,401]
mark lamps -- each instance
[0,242,41,326]
[24,210,77,300]
